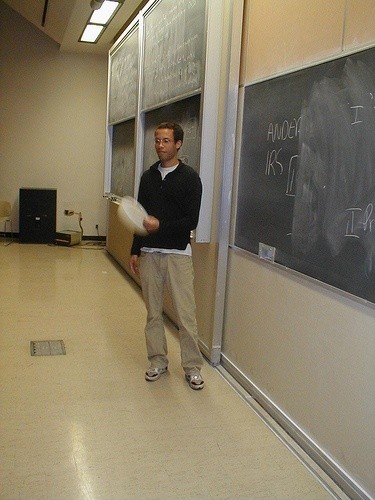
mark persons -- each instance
[128,122,206,390]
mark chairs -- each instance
[0,199,17,247]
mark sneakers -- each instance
[185,370,205,390]
[144,363,168,381]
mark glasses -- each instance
[152,139,179,146]
